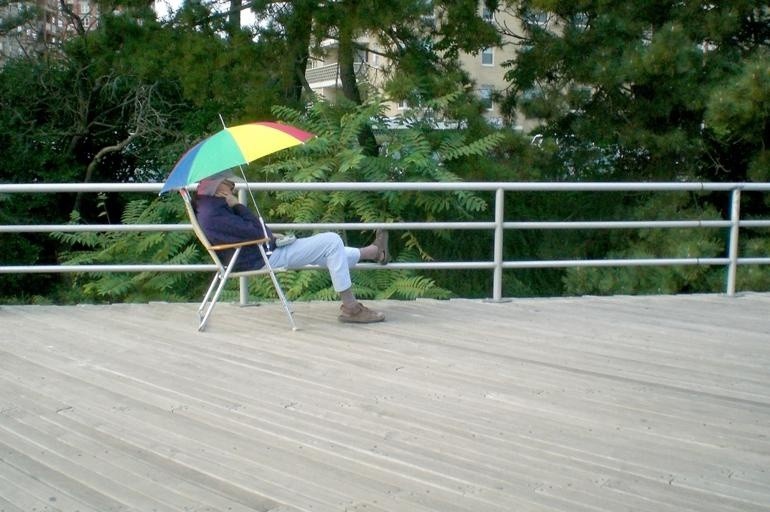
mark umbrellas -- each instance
[157,112,317,257]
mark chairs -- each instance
[178,187,300,333]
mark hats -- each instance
[197,171,246,196]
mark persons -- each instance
[194,168,390,323]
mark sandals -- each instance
[372,229,388,264]
[339,301,384,323]
